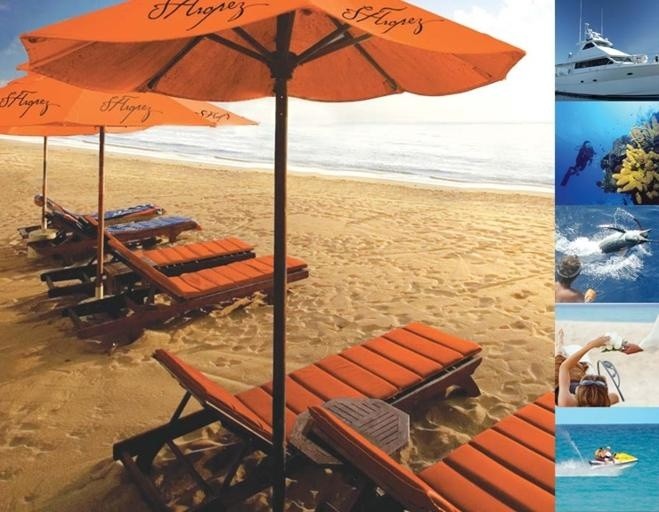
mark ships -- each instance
[556,22,658,98]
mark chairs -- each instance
[301,393,555,512]
[112,322,481,511]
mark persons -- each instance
[595,446,612,461]
[561,141,594,186]
[555,327,619,407]
[555,255,596,303]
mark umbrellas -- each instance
[0,74,259,299]
[17,0,525,511]
[0,126,152,231]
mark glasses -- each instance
[579,380,607,389]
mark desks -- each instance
[289,398,410,510]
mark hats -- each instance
[557,256,581,278]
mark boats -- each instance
[583,451,641,470]
